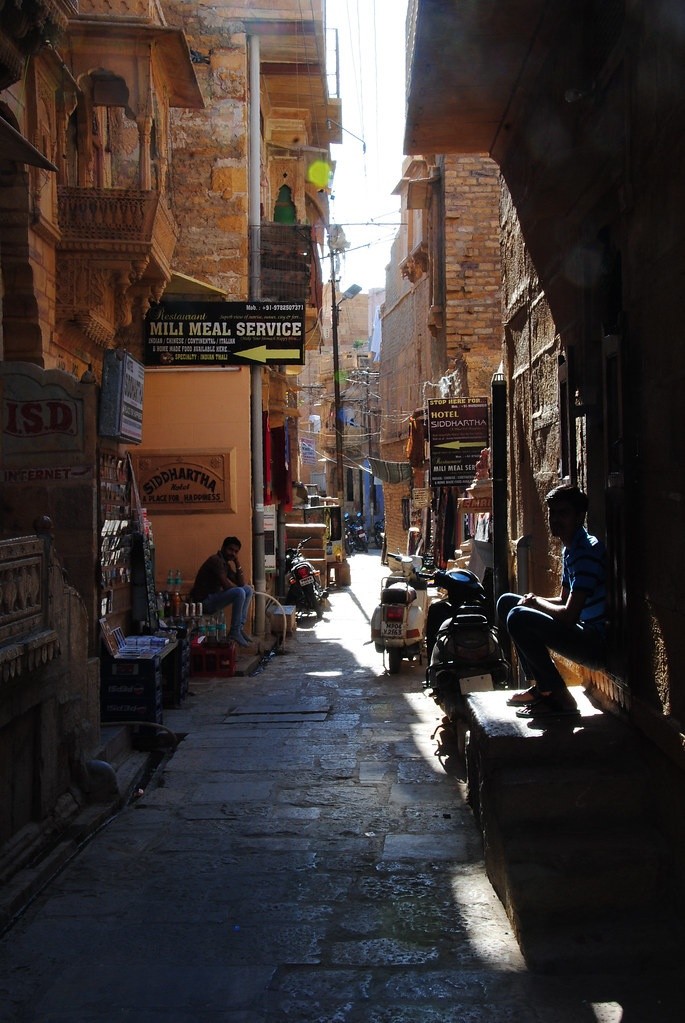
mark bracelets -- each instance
[238,571,243,576]
[237,566,242,570]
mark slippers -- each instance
[516,695,579,716]
[506,686,544,706]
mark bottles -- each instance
[217,610,226,642]
[169,616,178,632]
[207,615,217,642]
[166,570,175,592]
[157,592,164,618]
[173,593,182,616]
[197,615,207,637]
[175,569,183,591]
[163,592,173,617]
[187,619,198,639]
[178,615,187,638]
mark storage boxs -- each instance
[101,706,162,736]
[142,627,189,709]
[100,675,163,700]
[100,655,162,682]
[200,642,237,678]
[99,693,163,718]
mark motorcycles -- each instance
[374,519,384,547]
[345,527,354,555]
[345,512,369,553]
[285,536,325,619]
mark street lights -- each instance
[330,253,361,525]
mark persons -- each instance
[497,485,620,717]
[191,537,252,648]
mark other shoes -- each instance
[227,632,249,648]
[241,630,253,643]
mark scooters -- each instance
[371,548,434,673]
[415,568,508,721]
